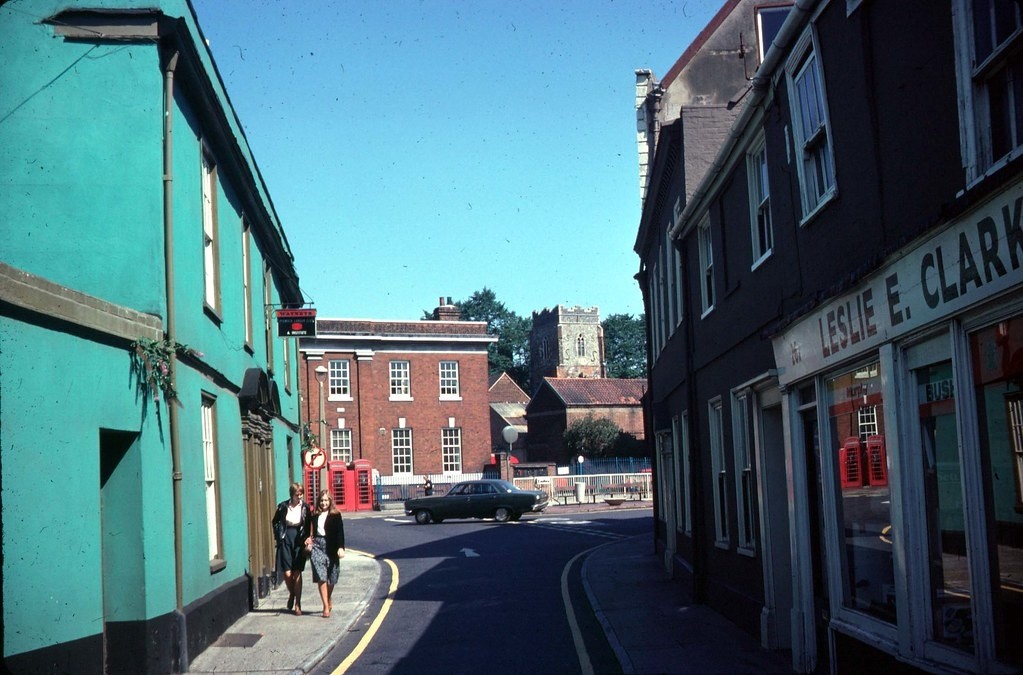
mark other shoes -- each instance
[323,607,330,618]
[295,600,301,615]
[287,593,295,609]
[328,597,332,610]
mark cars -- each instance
[405,479,549,524]
[625,468,653,491]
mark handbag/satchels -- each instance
[305,521,313,551]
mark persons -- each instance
[272,483,311,615]
[304,490,345,617]
[423,475,432,496]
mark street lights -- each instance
[578,456,584,483]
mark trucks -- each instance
[491,453,523,476]
